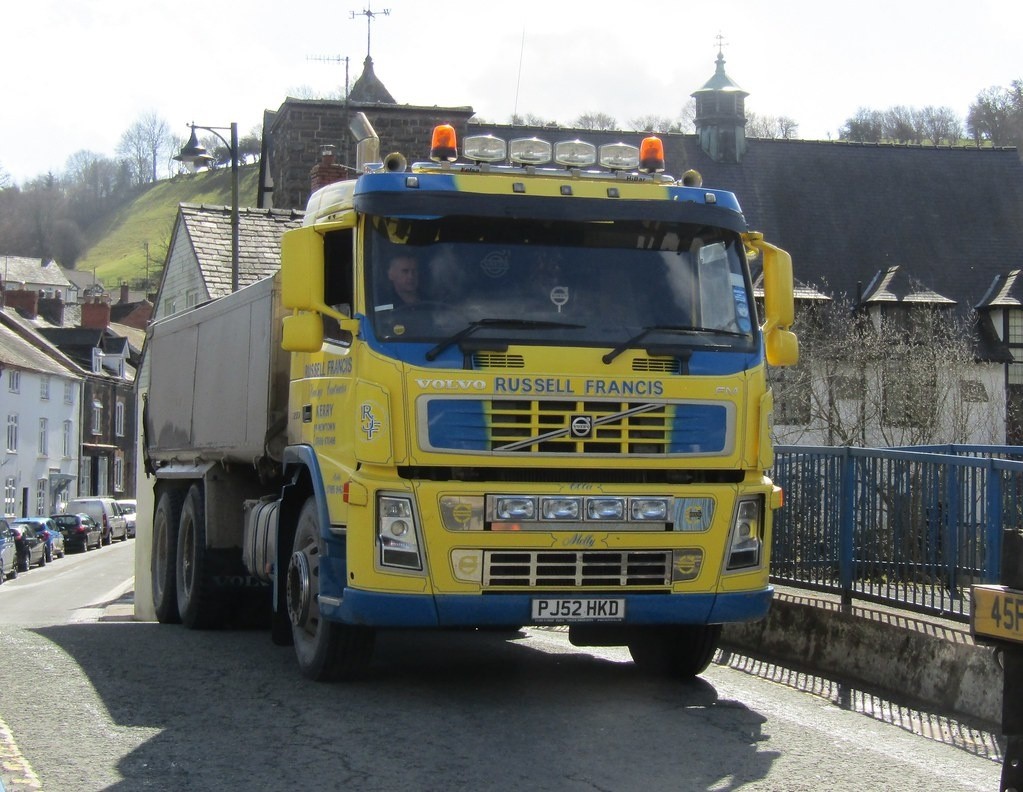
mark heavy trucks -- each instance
[137,124,802,679]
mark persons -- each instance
[372,255,442,314]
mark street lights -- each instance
[173,119,240,292]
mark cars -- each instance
[10,523,48,571]
[0,516,20,584]
[116,499,136,537]
[47,513,102,553]
[12,516,66,562]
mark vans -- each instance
[65,497,128,546]
